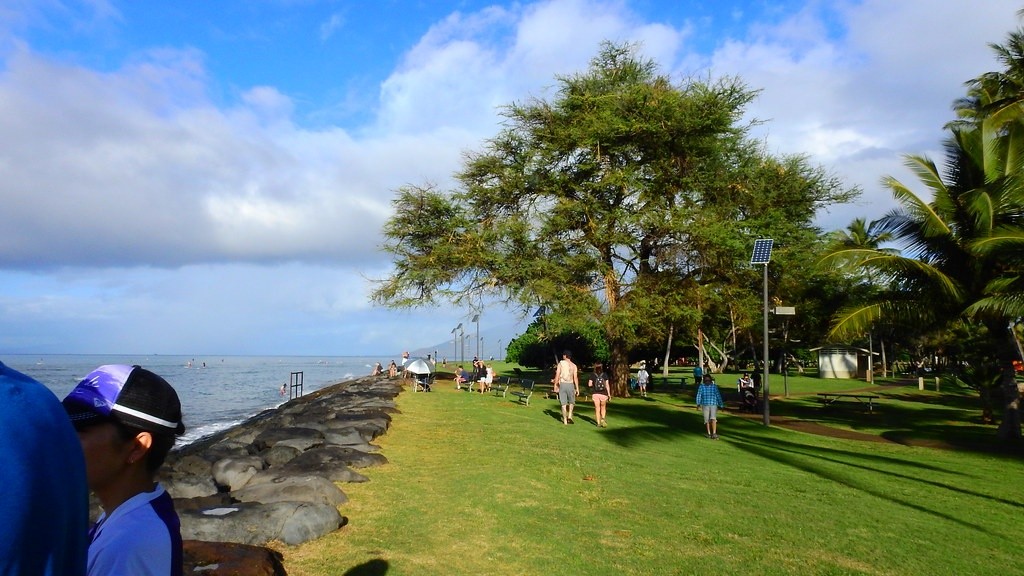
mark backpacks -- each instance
[594,373,606,391]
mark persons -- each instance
[418,374,430,392]
[553,350,579,425]
[695,374,724,440]
[281,384,287,394]
[738,373,756,406]
[472,356,494,394]
[389,360,397,377]
[453,366,469,390]
[0,359,185,576]
[588,362,612,428]
[638,368,649,397]
[751,369,762,397]
[694,364,702,384]
[402,352,409,371]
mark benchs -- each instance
[502,377,534,407]
[816,392,879,415]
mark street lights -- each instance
[750,237,774,429]
[480,337,483,361]
[457,323,471,362]
[472,315,479,360]
[451,328,456,362]
[498,339,502,361]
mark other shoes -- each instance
[597,424,601,427]
[712,433,719,439]
[568,418,574,424]
[601,418,607,427]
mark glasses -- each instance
[704,380,712,382]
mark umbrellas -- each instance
[403,356,435,374]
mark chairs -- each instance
[414,376,431,393]
[463,374,476,392]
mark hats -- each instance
[57,364,186,436]
